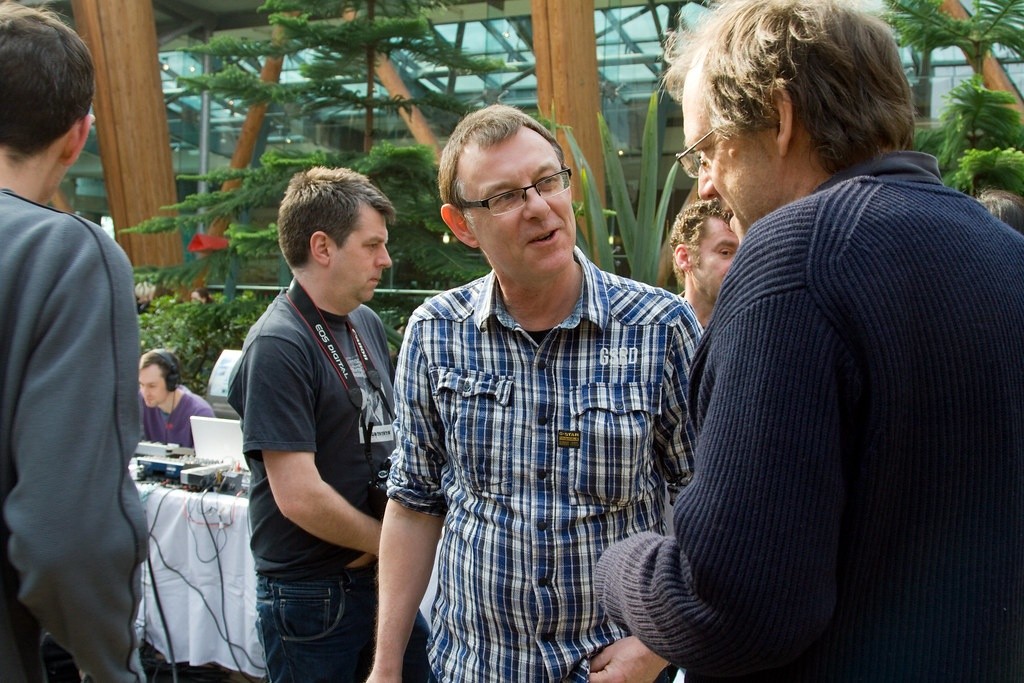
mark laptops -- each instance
[189,416,251,471]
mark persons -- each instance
[665,193,740,329]
[223,163,441,683]
[365,104,706,683]
[137,347,215,447]
[589,0,1024,683]
[0,1,163,683]
[963,186,1024,233]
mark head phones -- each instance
[149,348,180,392]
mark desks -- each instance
[130,459,266,677]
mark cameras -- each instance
[364,457,392,519]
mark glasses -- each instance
[675,126,717,179]
[456,164,572,216]
